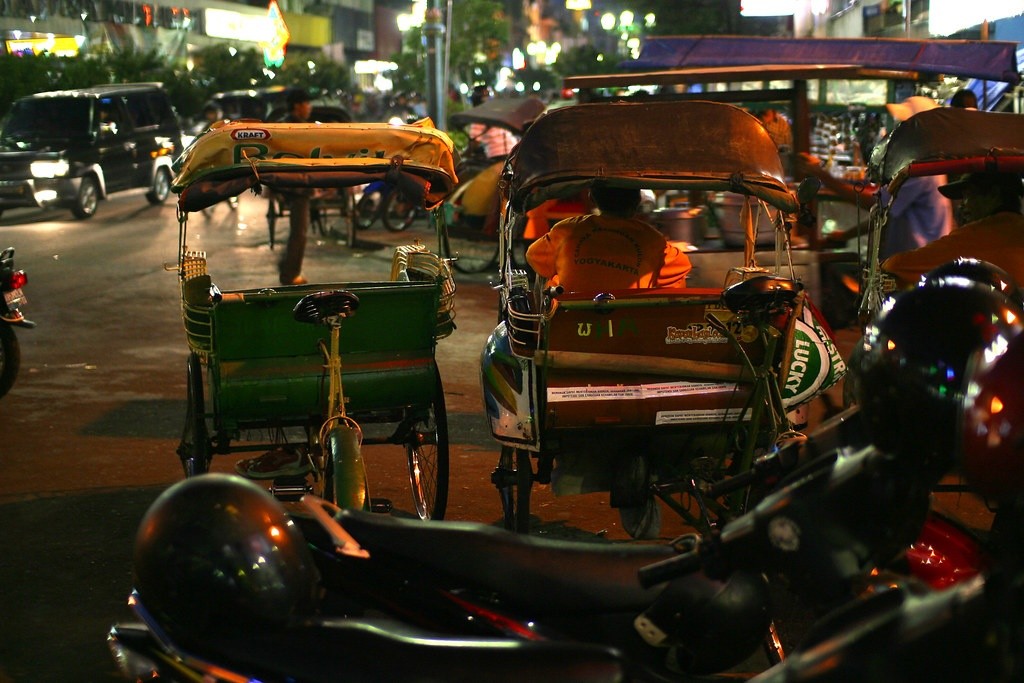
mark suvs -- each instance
[0,76,178,226]
[263,98,885,320]
[180,85,306,159]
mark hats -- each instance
[886,96,940,122]
[287,87,321,103]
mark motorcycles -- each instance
[107,386,1024,683]
[0,247,38,404]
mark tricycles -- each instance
[477,99,804,532]
[807,104,1024,326]
[168,117,460,521]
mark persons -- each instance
[201,99,234,130]
[525,177,692,298]
[278,88,319,286]
[951,88,978,110]
[878,169,1024,290]
[877,95,954,262]
[99,102,120,135]
